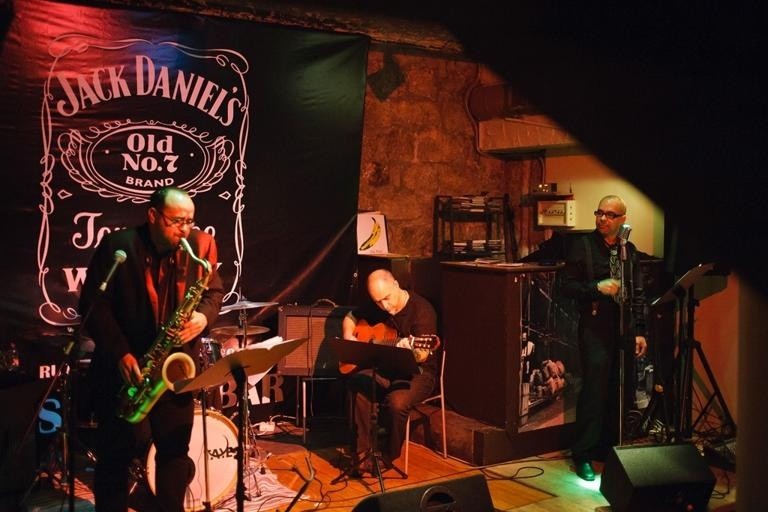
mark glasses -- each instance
[593,210,626,219]
[153,208,196,227]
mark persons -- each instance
[77,184,226,511]
[557,195,646,481]
[341,268,438,479]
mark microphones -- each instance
[98,249,127,292]
[615,223,632,263]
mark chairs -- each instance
[396,350,447,478]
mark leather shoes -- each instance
[574,458,596,482]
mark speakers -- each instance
[351,474,496,512]
[664,222,730,277]
[598,441,718,512]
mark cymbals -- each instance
[211,325,270,337]
[219,301,280,311]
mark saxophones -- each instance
[111,237,213,425]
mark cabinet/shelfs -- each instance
[433,191,515,264]
[439,258,566,431]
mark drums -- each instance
[137,400,239,512]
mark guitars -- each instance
[337,312,440,376]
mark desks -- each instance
[296,377,357,445]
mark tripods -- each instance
[330,366,409,497]
[633,279,737,438]
